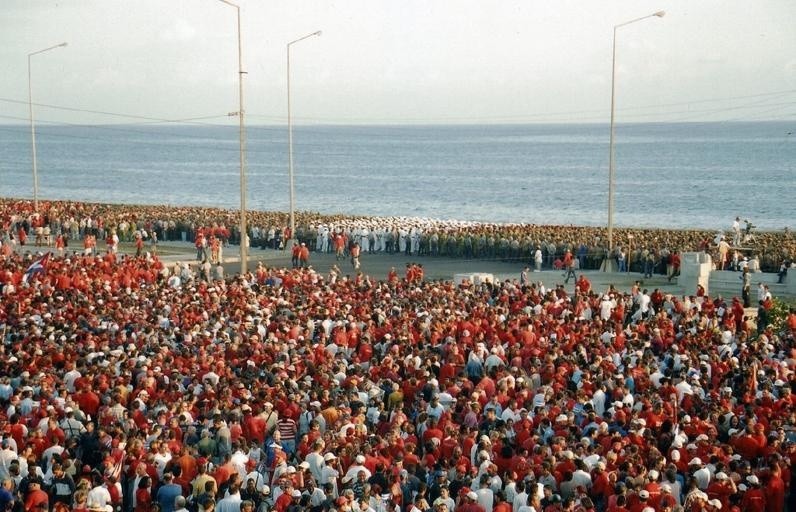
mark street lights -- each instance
[28,42,68,212]
[288,29,321,239]
[607,11,665,259]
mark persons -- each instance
[1,196,796,512]
